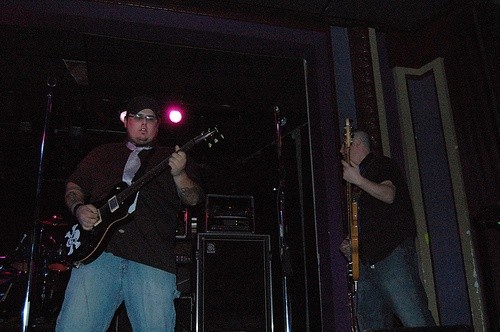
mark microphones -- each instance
[274,106,286,126]
[47,80,56,86]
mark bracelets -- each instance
[74,203,85,217]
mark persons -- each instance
[341,130,432,332]
[52,95,207,332]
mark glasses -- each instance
[126,114,158,123]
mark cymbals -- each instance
[36,217,69,226]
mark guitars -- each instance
[340,116,362,281]
[57,124,227,268]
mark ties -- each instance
[123,141,152,214]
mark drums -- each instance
[36,244,72,281]
[5,248,37,276]
[0,256,18,278]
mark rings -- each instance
[177,149,182,153]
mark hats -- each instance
[127,96,157,115]
